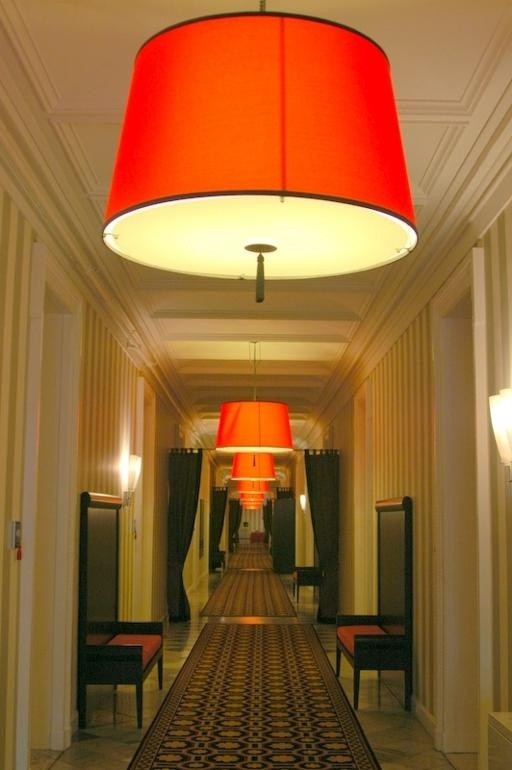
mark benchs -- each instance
[81,621,165,730]
[333,614,411,711]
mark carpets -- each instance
[127,623,383,770]
[225,541,274,569]
[198,570,296,618]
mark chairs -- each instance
[293,566,323,604]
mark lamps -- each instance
[485,387,512,473]
[118,453,144,509]
[99,0,418,308]
[213,341,298,511]
